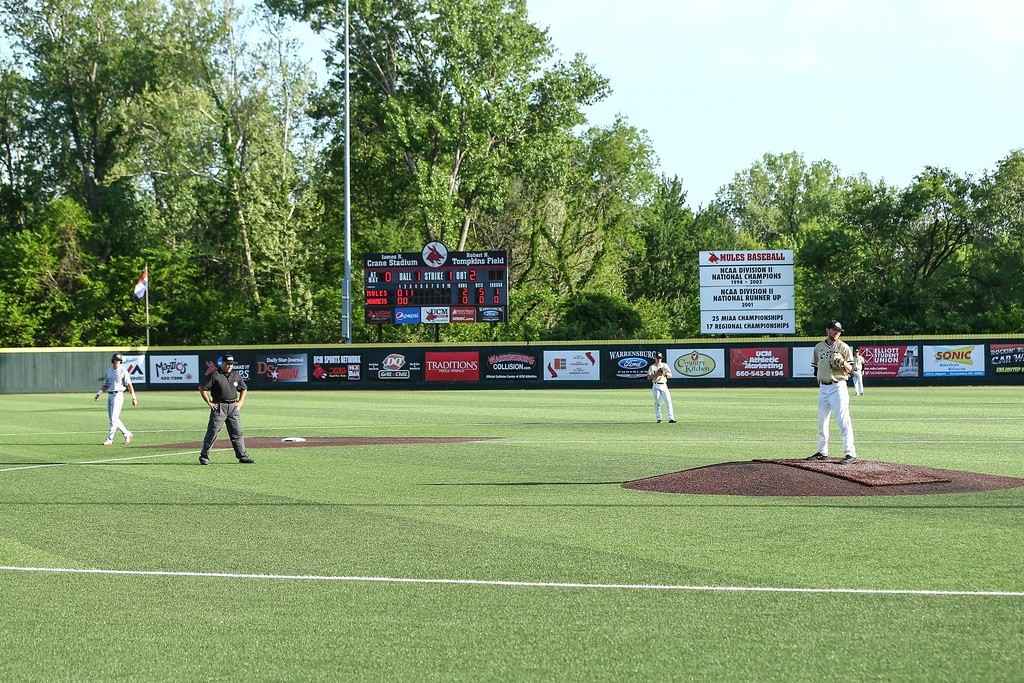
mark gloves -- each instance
[95,393,100,401]
[132,399,137,406]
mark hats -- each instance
[855,348,859,352]
[223,354,235,365]
[656,352,664,359]
[827,320,845,332]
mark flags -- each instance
[134,271,147,299]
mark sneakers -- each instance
[840,455,856,464]
[807,451,827,460]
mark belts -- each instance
[820,381,838,385]
[108,391,117,394]
[654,382,665,384]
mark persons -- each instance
[851,349,866,396]
[647,352,677,423]
[95,353,137,445]
[199,354,254,464]
[805,321,857,464]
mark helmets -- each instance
[111,354,122,363]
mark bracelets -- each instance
[98,390,103,396]
[132,393,136,400]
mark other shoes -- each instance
[201,457,209,466]
[240,458,254,463]
[125,432,133,444]
[102,438,112,445]
[669,420,676,423]
[658,420,663,423]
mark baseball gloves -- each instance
[658,367,668,376]
[830,352,843,370]
